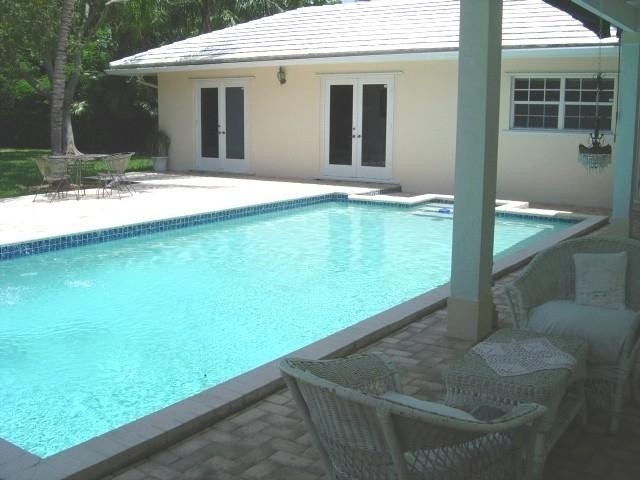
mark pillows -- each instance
[572,250,628,308]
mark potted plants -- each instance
[151,130,171,171]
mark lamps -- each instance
[575,18,614,171]
[277,64,287,84]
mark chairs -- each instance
[275,350,547,478]
[28,148,134,204]
[489,236,640,436]
